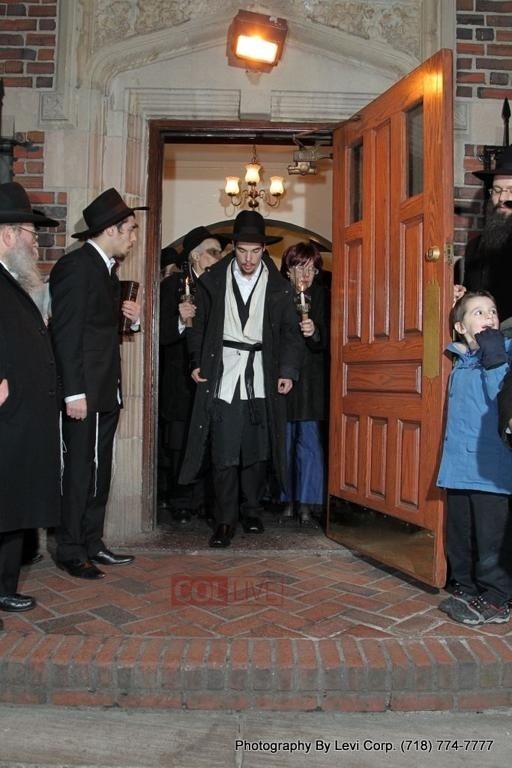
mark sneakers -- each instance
[438,592,510,624]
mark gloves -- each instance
[474,329,507,368]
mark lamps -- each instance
[222,10,289,72]
[224,140,287,210]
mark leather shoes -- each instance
[88,548,134,566]
[56,560,106,579]
[174,509,310,546]
[0,593,33,612]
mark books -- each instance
[115,279,139,334]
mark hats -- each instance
[160,210,283,267]
[0,183,60,226]
[71,188,150,238]
[472,152,512,180]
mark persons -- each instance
[453,149,510,441]
[49,188,143,579]
[0,180,60,629]
[435,288,512,624]
[162,210,331,546]
